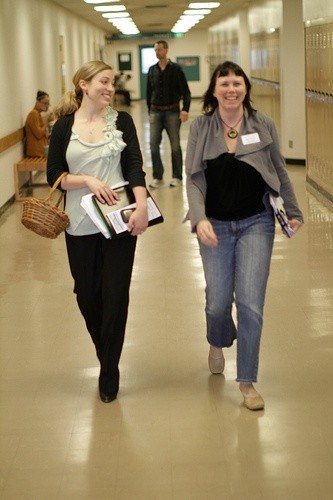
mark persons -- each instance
[112,67,136,110]
[148,40,191,188]
[45,62,148,404]
[185,60,303,409]
[24,91,59,159]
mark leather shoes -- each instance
[98,368,120,403]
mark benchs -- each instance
[14,156,54,202]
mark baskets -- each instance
[22,171,70,240]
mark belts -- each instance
[151,104,180,109]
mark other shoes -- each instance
[208,345,225,375]
[239,383,265,410]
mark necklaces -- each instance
[80,112,101,135]
[218,112,242,139]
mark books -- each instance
[267,194,299,238]
[80,181,163,240]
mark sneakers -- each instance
[169,177,182,187]
[148,179,163,188]
